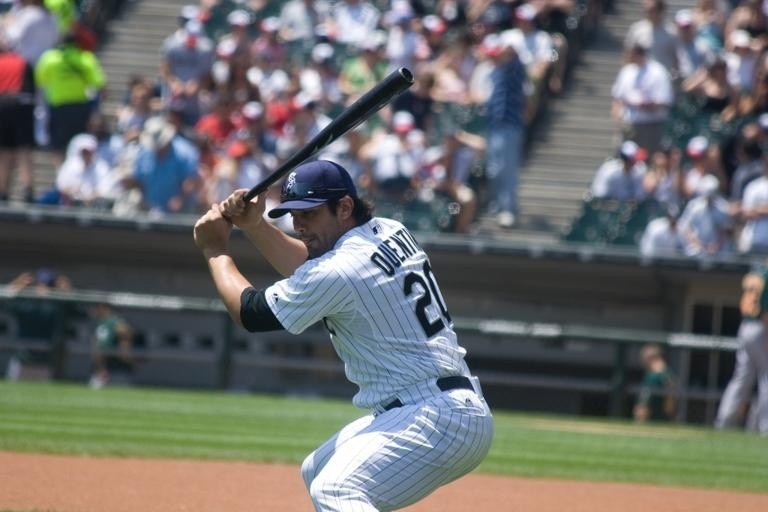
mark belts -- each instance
[373,375,476,418]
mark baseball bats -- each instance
[244,65,414,201]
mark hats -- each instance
[179,0,536,182]
[268,160,356,219]
[140,115,178,152]
[620,7,763,170]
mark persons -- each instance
[193,159,494,511]
